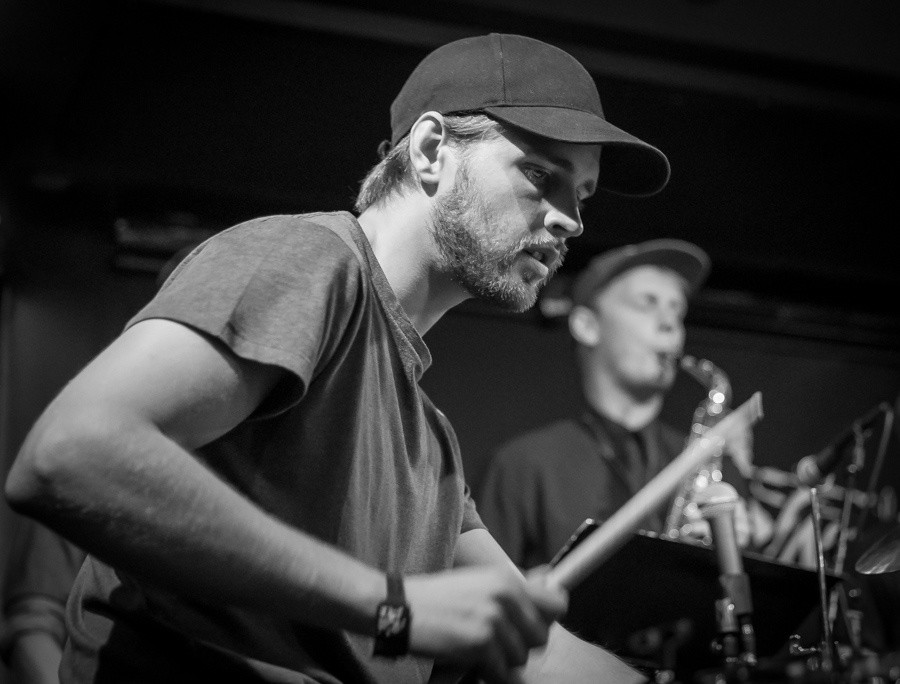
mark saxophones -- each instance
[651,351,734,545]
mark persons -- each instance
[3,31,672,683]
[477,236,712,567]
[0,242,207,684]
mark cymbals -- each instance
[854,526,900,575]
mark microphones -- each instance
[698,480,759,664]
[795,401,888,489]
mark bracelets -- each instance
[372,568,411,660]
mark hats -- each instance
[565,239,712,304]
[389,33,669,201]
